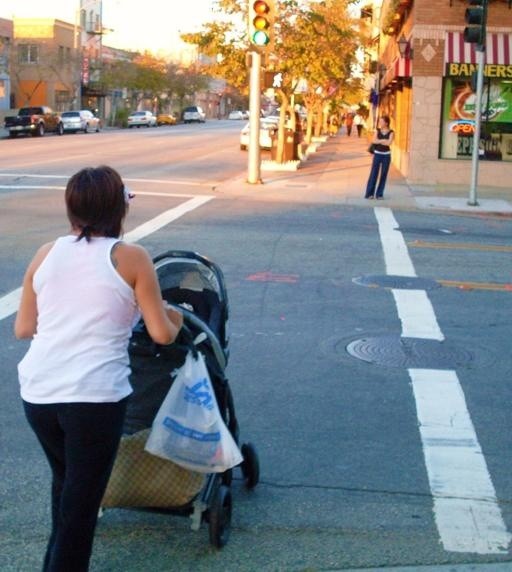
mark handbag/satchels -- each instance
[144,351,247,474]
[367,144,376,154]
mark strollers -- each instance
[96,250,260,548]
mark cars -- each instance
[228,107,281,151]
[4,105,206,137]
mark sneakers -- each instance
[367,195,384,200]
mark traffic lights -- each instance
[463,7,487,52]
[248,0,274,48]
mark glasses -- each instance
[125,192,137,202]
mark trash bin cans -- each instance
[269,127,295,162]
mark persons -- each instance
[14,164,184,571]
[365,116,394,198]
[329,112,367,137]
